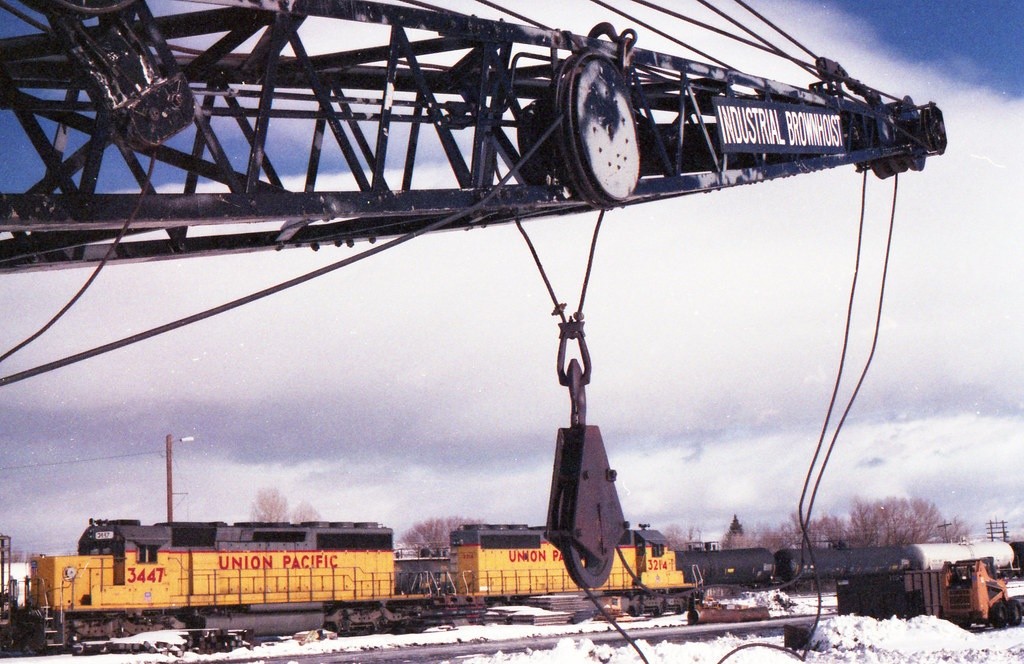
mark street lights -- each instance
[166,434,195,523]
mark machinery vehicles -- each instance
[941,556,1024,630]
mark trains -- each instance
[675,538,1024,596]
[10,518,704,654]
